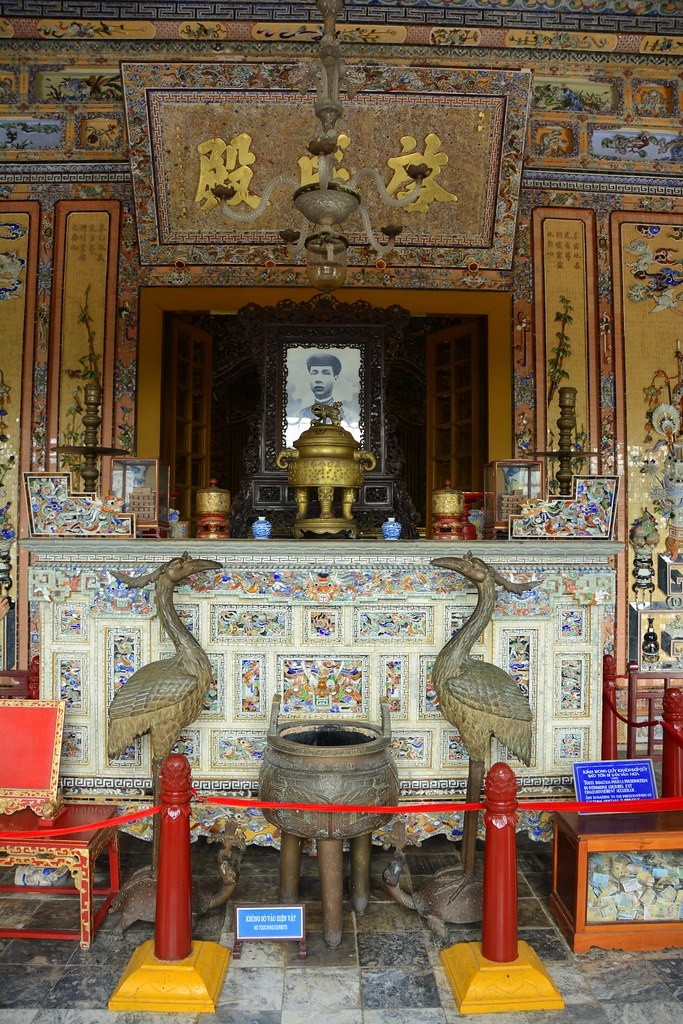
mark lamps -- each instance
[211,0,433,294]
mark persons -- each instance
[301,353,345,423]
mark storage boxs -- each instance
[548,811,683,954]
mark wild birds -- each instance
[429,549,543,905]
[107,551,224,890]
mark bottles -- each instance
[251,517,271,539]
[381,517,402,541]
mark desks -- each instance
[0,804,122,952]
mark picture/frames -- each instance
[274,337,371,460]
